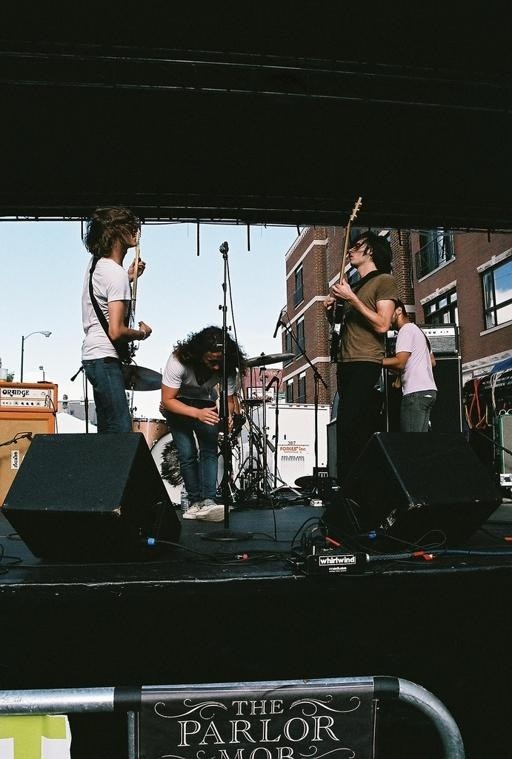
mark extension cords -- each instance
[290,546,335,569]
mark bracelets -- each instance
[142,330,147,340]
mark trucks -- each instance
[242,402,332,510]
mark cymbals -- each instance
[246,353,296,367]
[120,365,163,391]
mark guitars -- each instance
[119,217,140,363]
[330,197,362,362]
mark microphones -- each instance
[273,311,283,338]
[265,375,278,392]
[218,241,229,253]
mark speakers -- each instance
[318,431,503,553]
[1,432,182,563]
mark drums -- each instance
[150,431,225,508]
[132,418,168,450]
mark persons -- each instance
[322,230,400,484]
[80,205,152,432]
[382,299,439,433]
[160,326,248,522]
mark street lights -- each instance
[21,329,51,383]
[39,366,46,382]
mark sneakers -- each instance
[195,502,230,523]
[182,502,201,520]
